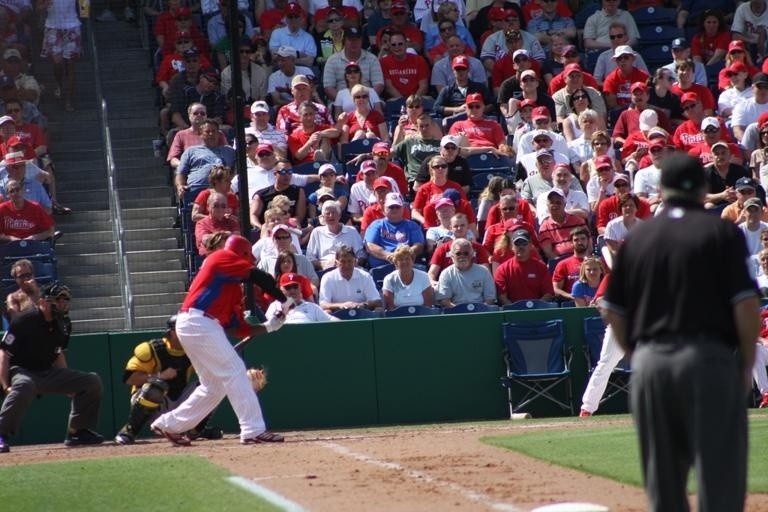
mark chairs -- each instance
[584,319,630,411]
[177,176,213,290]
[573,1,705,83]
[286,93,516,211]
[333,300,580,319]
[2,239,55,294]
[502,319,577,421]
[548,252,598,276]
[319,263,428,289]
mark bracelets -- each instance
[364,301,371,312]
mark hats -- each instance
[279,272,302,286]
[250,100,270,114]
[648,142,667,155]
[389,2,408,16]
[7,136,26,147]
[520,69,537,84]
[443,188,461,202]
[724,61,747,76]
[284,2,301,15]
[373,178,391,193]
[434,199,455,208]
[700,116,720,129]
[681,92,699,105]
[531,129,555,142]
[594,154,612,171]
[439,133,460,149]
[552,161,572,173]
[274,45,298,60]
[465,94,483,105]
[512,229,530,243]
[671,37,691,50]
[752,72,768,85]
[505,29,522,42]
[488,7,504,22]
[563,63,582,78]
[0,116,15,128]
[611,46,638,65]
[711,140,729,152]
[372,142,392,155]
[292,74,311,90]
[183,47,201,58]
[630,82,649,95]
[744,197,763,211]
[317,163,337,176]
[344,26,364,38]
[519,98,537,109]
[638,109,658,131]
[174,6,191,18]
[271,223,290,239]
[758,111,768,126]
[728,40,746,55]
[360,160,376,174]
[384,192,404,207]
[532,106,550,120]
[344,61,361,71]
[502,9,519,20]
[660,152,706,191]
[504,217,524,231]
[316,186,336,199]
[561,45,581,58]
[0,151,32,165]
[612,174,630,187]
[534,149,554,158]
[256,143,274,154]
[3,48,22,63]
[0,75,14,90]
[735,177,755,190]
[513,49,530,63]
[452,55,469,71]
[174,31,193,40]
[546,187,567,200]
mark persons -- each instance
[428,213,492,290]
[587,156,625,202]
[347,115,445,182]
[671,60,718,115]
[435,238,497,308]
[749,227,767,276]
[481,10,546,70]
[470,0,527,41]
[159,47,208,135]
[382,245,433,308]
[391,95,444,142]
[604,194,646,254]
[154,30,213,96]
[597,174,650,233]
[97,0,138,23]
[268,195,300,227]
[243,100,288,145]
[411,155,477,237]
[252,208,302,262]
[584,0,640,49]
[594,23,650,86]
[380,32,430,100]
[0,115,38,160]
[613,81,672,145]
[207,0,255,46]
[363,193,425,267]
[537,164,590,222]
[753,249,768,287]
[516,133,578,182]
[702,141,749,210]
[200,0,251,14]
[0,48,40,107]
[431,34,488,96]
[375,1,422,50]
[650,67,685,118]
[276,75,335,131]
[634,145,667,215]
[482,195,543,254]
[433,55,496,117]
[306,162,349,211]
[288,101,340,163]
[718,60,757,118]
[269,46,328,110]
[0,180,55,243]
[5,100,73,215]
[527,0,576,51]
[338,85,391,143]
[579,273,628,417]
[552,63,606,122]
[539,188,594,260]
[231,146,346,197]
[540,32,569,82]
[735,198,768,259]
[6,259,43,322]
[225,89,253,121]
[174,120,237,197]
[334,61,383,117]
[730,0,768,51]
[113,315,224,444]
[214,12,256,69]
[36,1,82,113]
[604,45,654,110]
[637,125,678,168]
[477,7,508,44]
[489,215,546,266]
[493,230,555,304]
[367,0,401,44]
[571,259,605,308]
[562,89,604,140]
[0,151,53,216]
[167,102,228,168]
[580,130,623,179]
[425,199,477,251]
[517,106,570,159]
[0,8,28,51]
[272,250,319,297]
[492,27,541,94]
[447,93,513,159]
[687,116,743,165]
[414,135,474,195]
[304,187,352,242]
[672,93,732,152]
[161,7,209,55]
[749,112,768,203]
[0,0,34,22]
[721,177,767,224]
[318,10,359,63]
[194,193,242,253]
[205,232,230,252]
[266,4,317,64]
[305,199,368,271]
[257,224,320,287]
[356,143,408,194]
[717,36,758,88]
[498,49,531,115]
[249,159,306,227]
[548,45,596,97]
[260,0,309,35]
[423,5,477,57]
[316,245,382,312]
[150,235,287,442]
[313,0,362,33]
[167,69,225,126]
[603,155,758,512]
[424,189,478,240]
[0,142,54,185]
[190,166,240,220]
[507,99,537,153]
[732,72,768,140]
[0,279,105,452]
[568,106,615,173]
[240,133,269,174]
[360,179,411,238]
[477,176,503,241]
[144,0,202,57]
[256,0,311,24]
[221,42,268,102]
[551,226,590,299]
[522,0,572,18]
[324,28,384,100]
[347,160,404,221]
[691,9,729,66]
[511,69,558,129]
[620,108,671,155]
[0,75,49,140]
[521,149,585,199]
[264,273,340,328]
[750,304,768,408]
[482,179,539,229]
[414,0,470,26]
[661,37,706,89]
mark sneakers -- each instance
[192,425,228,441]
[240,431,285,444]
[64,429,105,446]
[115,427,135,446]
[577,409,593,418]
[758,392,768,408]
[0,434,11,453]
[151,422,192,447]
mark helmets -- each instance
[224,235,256,264]
[167,314,179,330]
[39,280,71,326]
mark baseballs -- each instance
[234,302,296,352]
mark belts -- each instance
[655,328,697,338]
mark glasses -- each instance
[185,58,198,64]
[451,8,459,13]
[685,102,695,112]
[245,140,255,147]
[189,111,207,117]
[455,67,467,72]
[728,72,738,77]
[178,41,190,44]
[259,151,272,158]
[240,49,253,55]
[389,205,401,211]
[281,208,292,216]
[323,171,334,177]
[536,119,550,124]
[616,182,627,188]
[501,206,516,212]
[740,188,754,196]
[468,104,481,110]
[8,187,22,194]
[705,127,718,134]
[346,68,360,74]
[524,76,534,83]
[454,251,470,257]
[376,152,389,157]
[514,240,526,246]
[354,94,370,100]
[7,108,21,114]
[286,15,301,19]
[284,284,299,291]
[608,33,625,40]
[391,42,404,47]
[274,234,290,240]
[16,272,34,280]
[445,146,457,152]
[439,26,454,34]
[566,52,576,57]
[761,130,768,137]
[615,54,630,62]
[407,103,421,108]
[540,156,553,163]
[274,168,295,176]
[574,94,588,102]
[516,57,526,63]
[598,166,609,173]
[431,163,448,170]
[211,203,227,209]
[666,76,677,83]
[327,16,342,24]
[205,77,218,86]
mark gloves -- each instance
[248,369,265,392]
[279,297,296,314]
[265,313,285,330]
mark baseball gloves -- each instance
[246,369,266,391]
